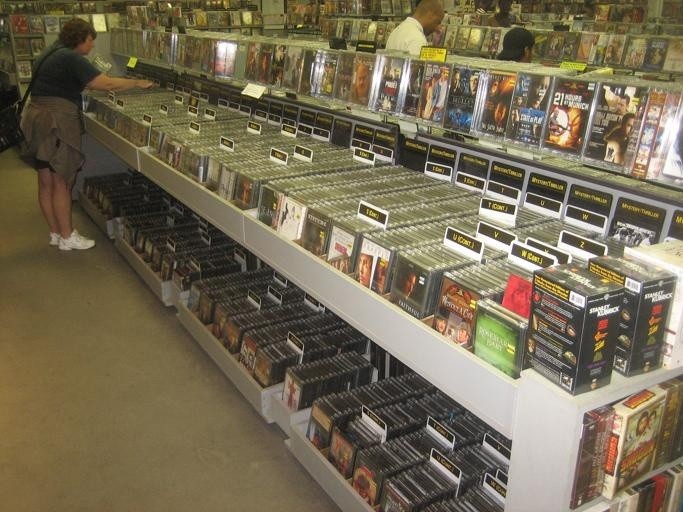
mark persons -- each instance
[422,66,636,166]
[18,17,159,251]
[384,0,445,56]
[356,222,471,352]
[495,27,535,63]
[356,64,368,98]
[383,58,393,77]
[625,407,657,454]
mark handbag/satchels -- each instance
[0,105,25,152]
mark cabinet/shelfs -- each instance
[1,1,266,103]
[45,2,682,512]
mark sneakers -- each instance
[58,229,96,251]
[50,231,62,245]
